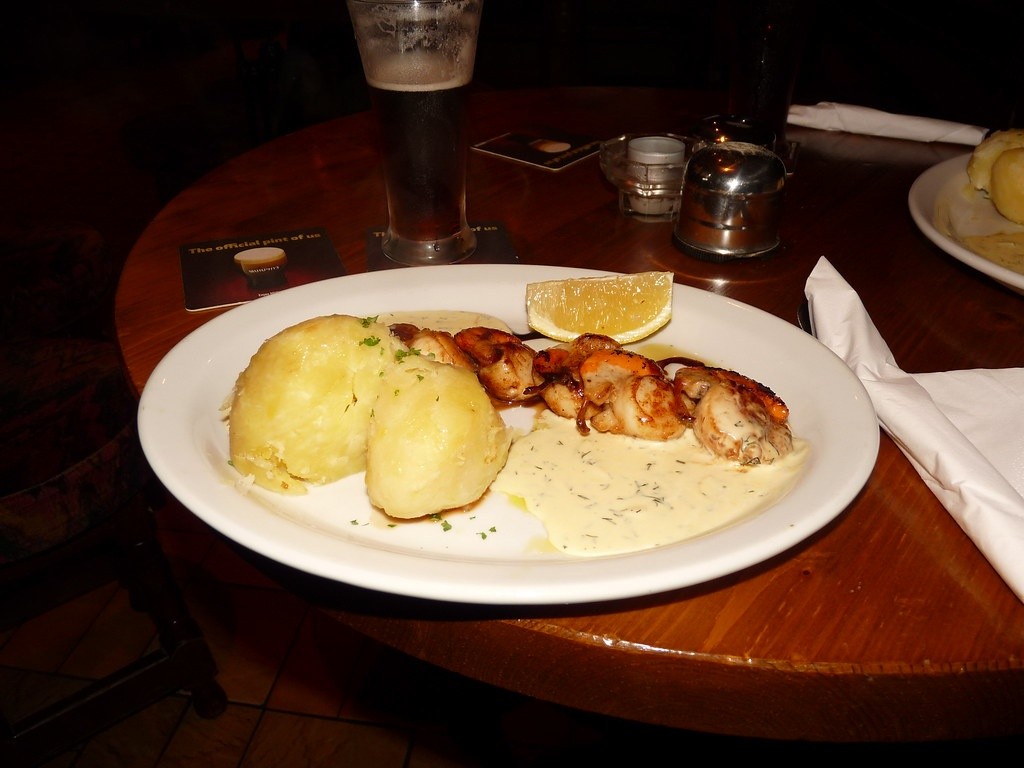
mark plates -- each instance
[137,260,883,605]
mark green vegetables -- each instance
[357,315,436,418]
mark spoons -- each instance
[797,302,812,334]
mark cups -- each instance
[234,247,288,289]
[907,149,1023,295]
[509,133,570,154]
[345,0,481,266]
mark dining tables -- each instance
[110,85,1024,741]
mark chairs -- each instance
[1,486,227,768]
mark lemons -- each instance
[525,268,674,344]
